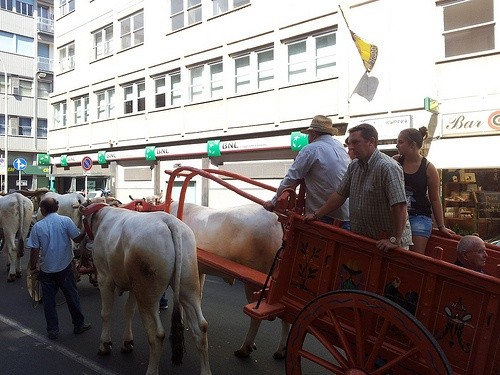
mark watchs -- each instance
[388,235,401,244]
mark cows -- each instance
[0,190,290,375]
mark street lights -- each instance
[83,171,91,199]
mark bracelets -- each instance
[438,224,445,229]
[312,210,319,220]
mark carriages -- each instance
[0,165,500,375]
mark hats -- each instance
[300,115,339,136]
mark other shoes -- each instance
[73,322,93,334]
[48,332,59,339]
[160,304,169,309]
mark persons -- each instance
[263,114,353,230]
[27,196,96,340]
[391,126,456,255]
[302,123,414,253]
[453,235,488,274]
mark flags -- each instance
[350,29,378,73]
